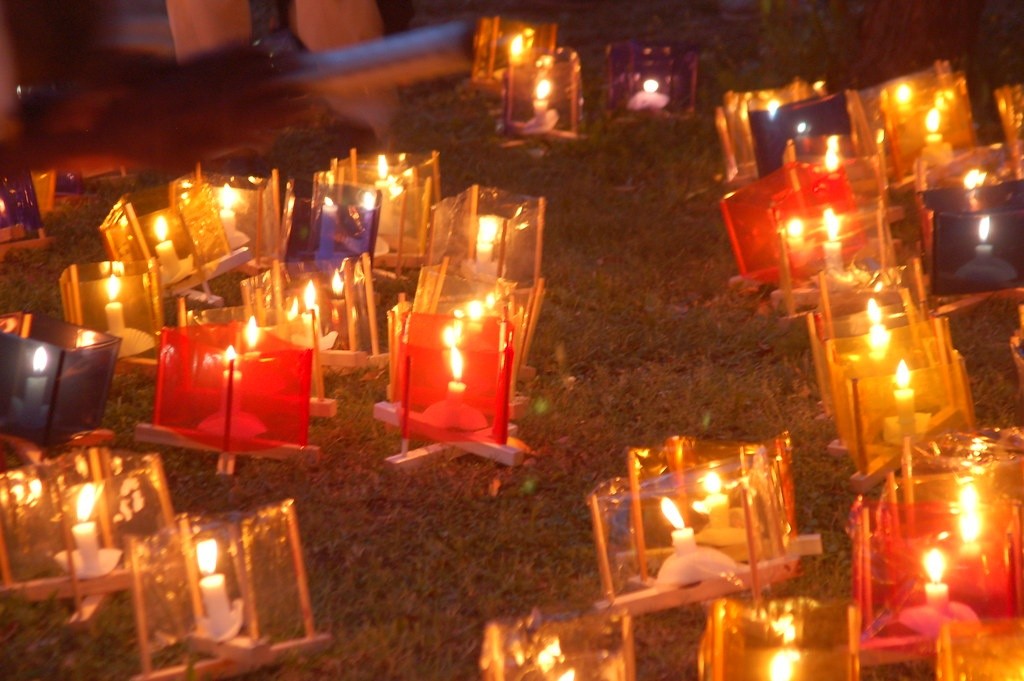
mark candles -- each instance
[19,34,992,681]
